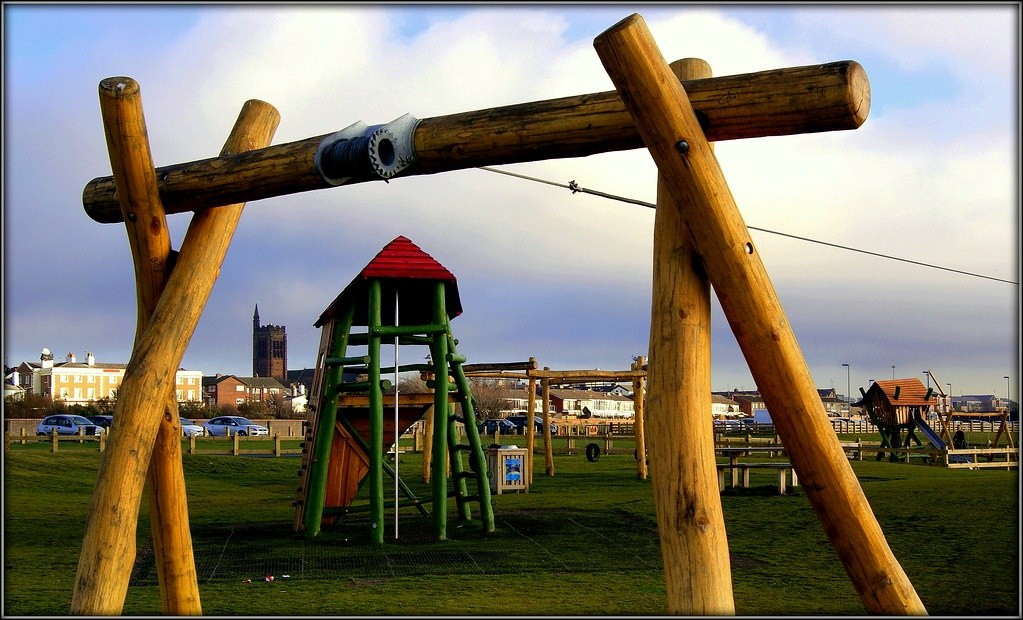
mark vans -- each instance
[507,416,556,436]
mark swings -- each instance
[468,375,522,449]
[957,416,971,457]
[978,414,991,459]
[567,378,618,462]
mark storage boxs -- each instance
[487,448,530,495]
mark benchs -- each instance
[714,447,799,496]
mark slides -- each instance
[912,416,973,464]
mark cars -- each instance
[36,415,105,443]
[477,419,517,435]
[89,415,114,432]
[179,417,208,437]
[203,416,269,436]
